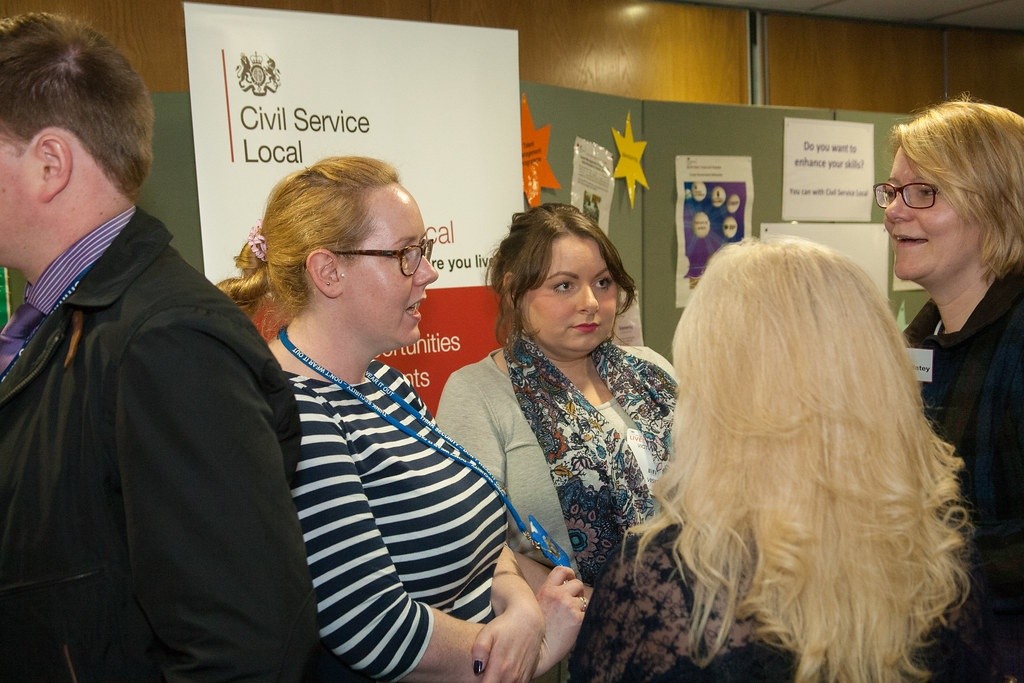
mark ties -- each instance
[0,303,46,376]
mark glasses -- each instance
[873,183,940,209]
[304,239,433,277]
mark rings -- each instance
[578,595,588,612]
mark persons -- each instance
[211,153,591,683]
[571,233,1001,683]
[0,9,323,683]
[437,200,681,683]
[868,100,1024,682]
